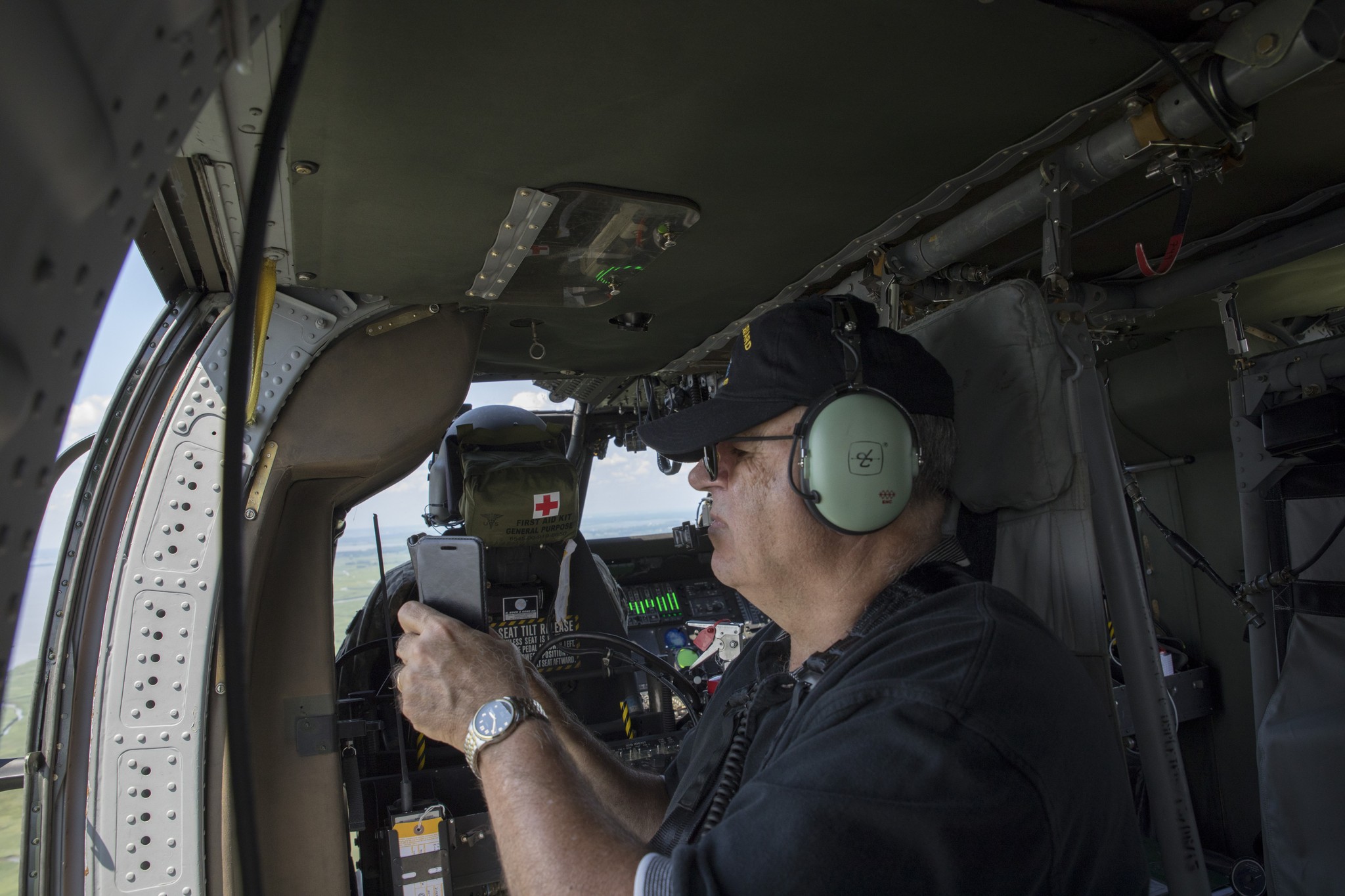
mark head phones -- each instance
[788,295,924,535]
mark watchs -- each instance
[465,695,549,778]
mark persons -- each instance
[393,294,1148,896]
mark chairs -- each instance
[363,421,633,751]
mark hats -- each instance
[635,293,957,464]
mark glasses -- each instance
[702,436,802,482]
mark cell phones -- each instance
[406,533,490,635]
